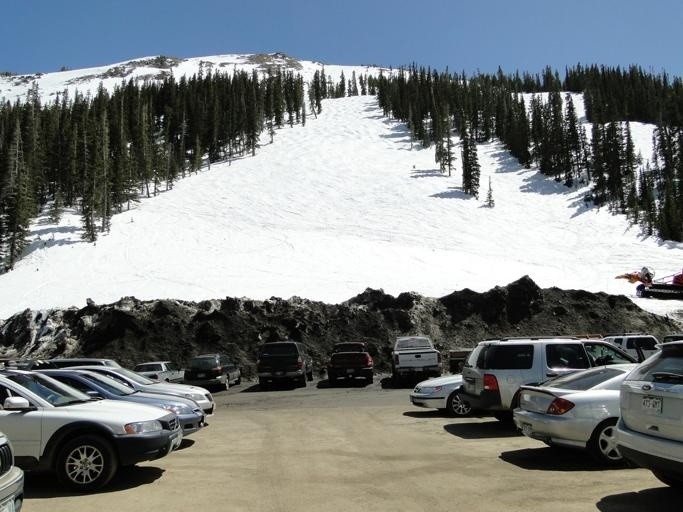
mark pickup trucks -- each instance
[327,343,373,383]
[391,337,442,380]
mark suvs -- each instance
[257,342,313,386]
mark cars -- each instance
[0,353,241,512]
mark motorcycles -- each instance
[636,268,683,299]
